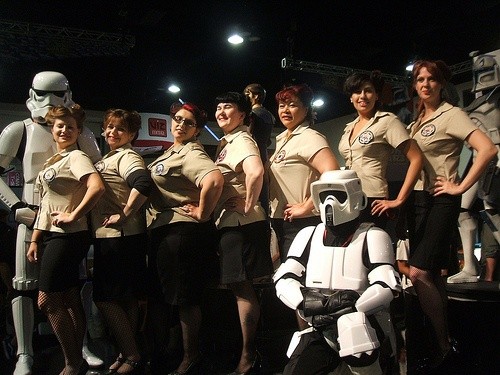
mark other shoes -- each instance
[230,349,262,375]
[174,355,197,374]
[108,357,125,370]
[117,360,139,374]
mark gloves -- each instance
[301,286,360,316]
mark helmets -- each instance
[310,168,369,227]
[26,71,75,126]
[470,54,500,92]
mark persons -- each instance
[212,91,274,375]
[0,71,103,375]
[270,224,281,273]
[264,79,340,332]
[244,84,274,215]
[90,109,157,375]
[447,50,500,283]
[144,99,224,375]
[338,71,424,375]
[479,219,498,282]
[396,229,413,289]
[404,61,498,370]
[27,104,105,375]
[272,167,403,375]
[0,215,48,361]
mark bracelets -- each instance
[30,241,39,245]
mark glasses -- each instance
[172,116,196,127]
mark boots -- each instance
[13,295,35,375]
[445,218,481,282]
[81,332,104,367]
